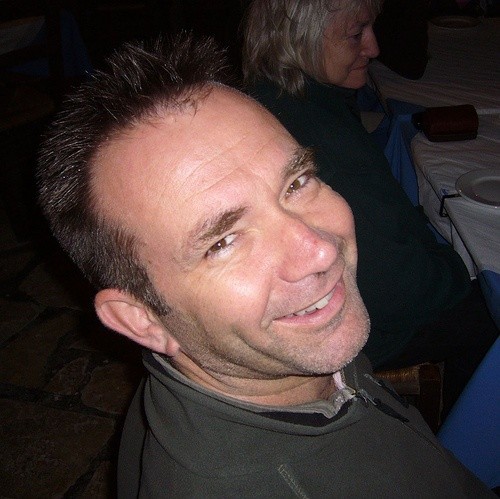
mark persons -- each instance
[243,1,500,433]
[36,29,499,499]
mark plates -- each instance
[430,16,479,31]
[455,168,500,207]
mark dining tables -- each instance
[367,17,500,332]
